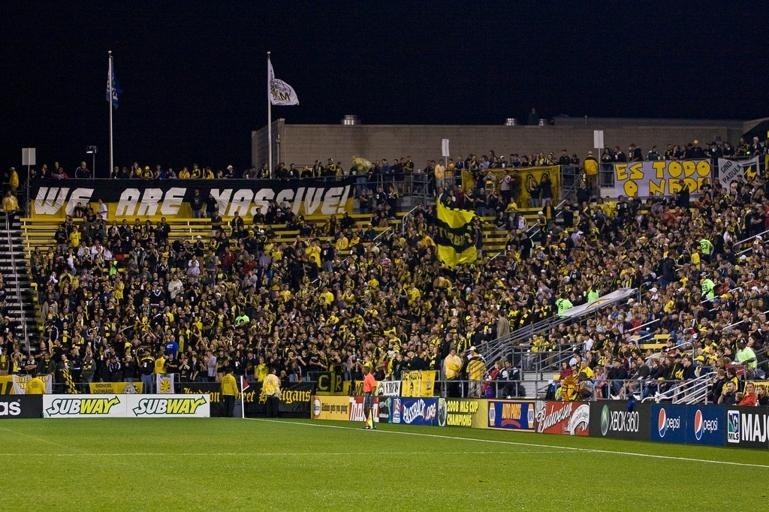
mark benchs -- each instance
[17,203,769,353]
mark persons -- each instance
[262,366,284,419]
[358,362,378,430]
[220,367,239,417]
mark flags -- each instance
[269,59,301,106]
[105,56,123,111]
